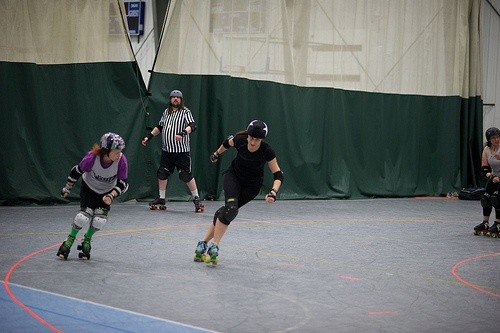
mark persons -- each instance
[474,127,500,233]
[61,132,129,252]
[194,119,284,256]
[143,90,202,208]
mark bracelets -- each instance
[272,189,277,194]
[182,130,187,135]
[489,175,493,179]
[216,150,222,156]
[148,133,154,139]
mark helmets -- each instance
[100,133,125,150]
[246,120,268,139]
[169,90,183,98]
[484,127,499,137]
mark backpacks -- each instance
[458,187,486,201]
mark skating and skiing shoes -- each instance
[193,241,208,262]
[192,195,205,213]
[149,195,167,210]
[487,220,500,238]
[205,242,220,264]
[57,235,78,260]
[78,234,92,260]
[473,220,489,235]
